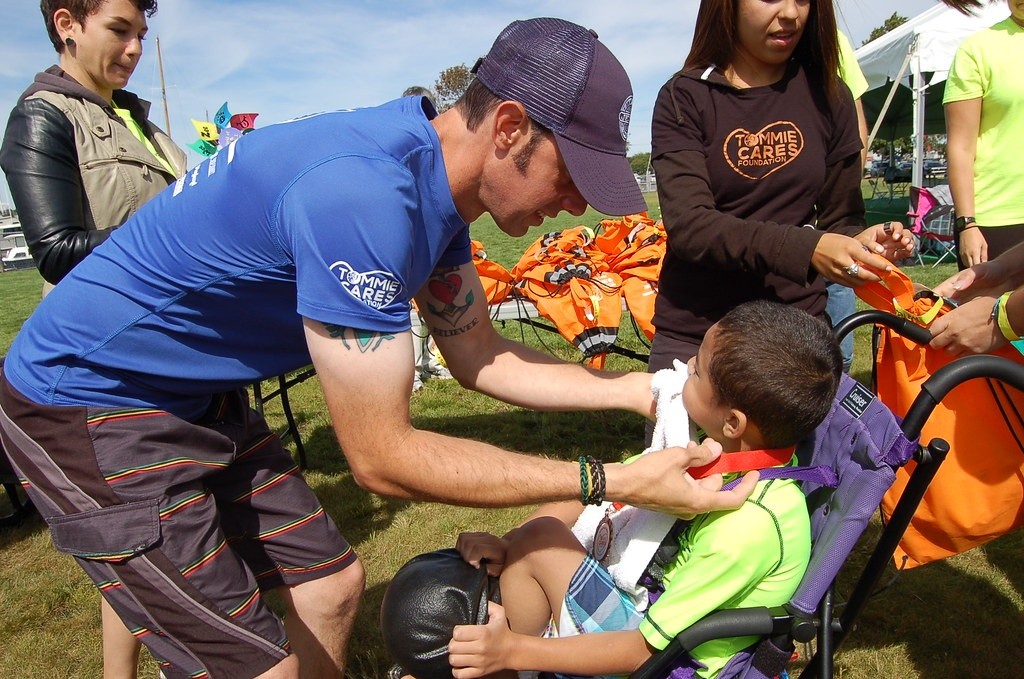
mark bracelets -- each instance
[959,226,977,234]
[578,455,605,507]
[992,291,1022,341]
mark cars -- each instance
[924,160,947,177]
[898,163,926,180]
[634,174,655,183]
[870,161,889,177]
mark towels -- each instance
[570,358,700,612]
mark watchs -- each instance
[955,216,975,231]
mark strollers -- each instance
[625,310,1024,679]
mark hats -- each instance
[470,17,648,217]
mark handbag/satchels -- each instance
[850,255,1024,571]
[469,208,668,368]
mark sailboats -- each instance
[0,168,22,238]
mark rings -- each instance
[884,222,892,235]
[846,264,859,278]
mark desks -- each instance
[884,179,909,203]
[253,297,649,470]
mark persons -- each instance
[645,0,915,448]
[448,301,840,679]
[0,0,186,679]
[942,0,1024,271]
[0,17,759,679]
[929,241,1024,357]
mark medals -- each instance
[593,517,612,560]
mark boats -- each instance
[0,234,37,268]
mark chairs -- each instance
[869,180,890,200]
[632,310,1024,679]
[907,185,958,271]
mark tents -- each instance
[854,0,1013,266]
